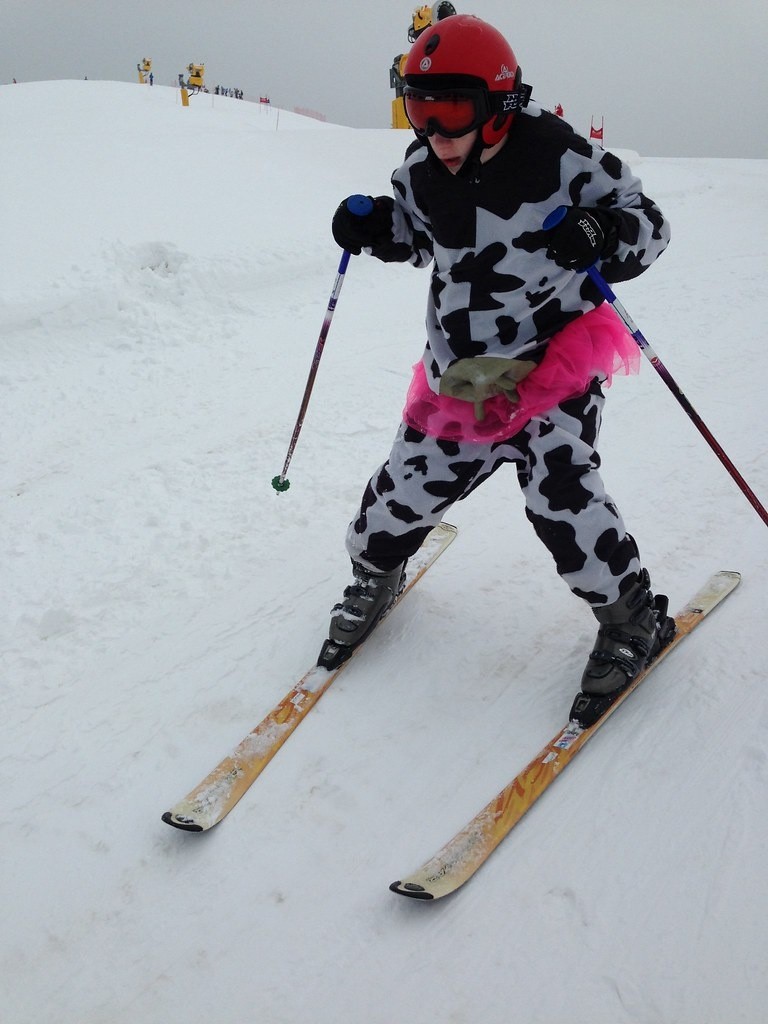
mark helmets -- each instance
[404,15,535,145]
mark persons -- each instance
[328,16,673,691]
[148,73,244,100]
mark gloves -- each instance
[545,205,627,274]
[331,195,412,262]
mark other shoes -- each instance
[581,601,690,697]
[328,563,404,645]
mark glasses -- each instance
[399,86,523,139]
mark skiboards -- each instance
[158,518,744,902]
